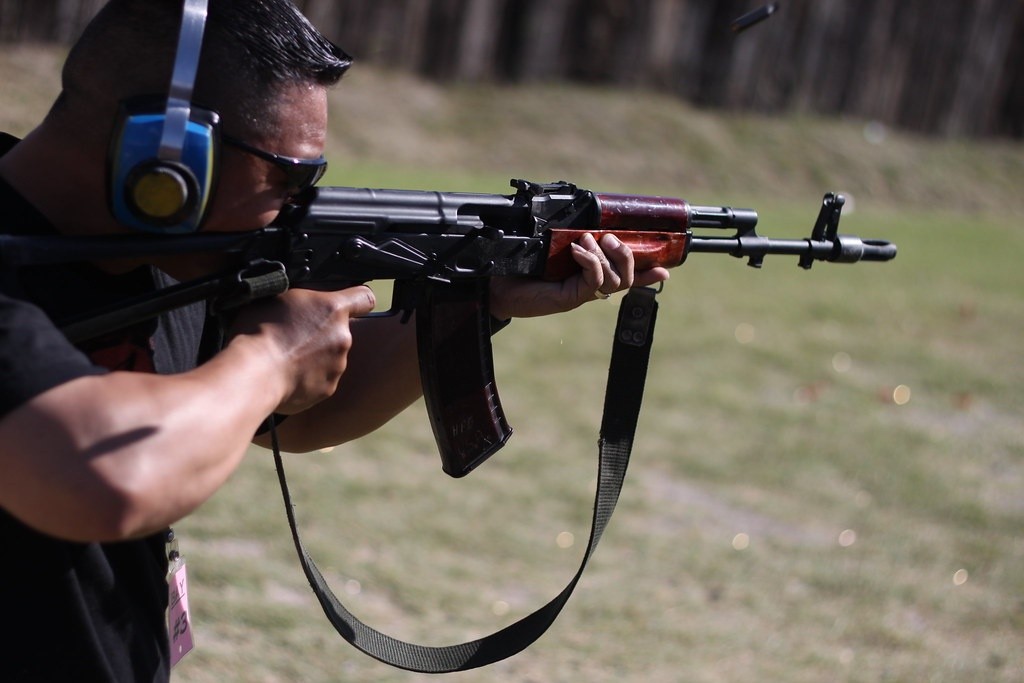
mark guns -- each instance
[255,180,895,477]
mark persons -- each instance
[0,1,671,683]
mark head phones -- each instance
[104,0,220,236]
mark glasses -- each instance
[215,133,328,198]
